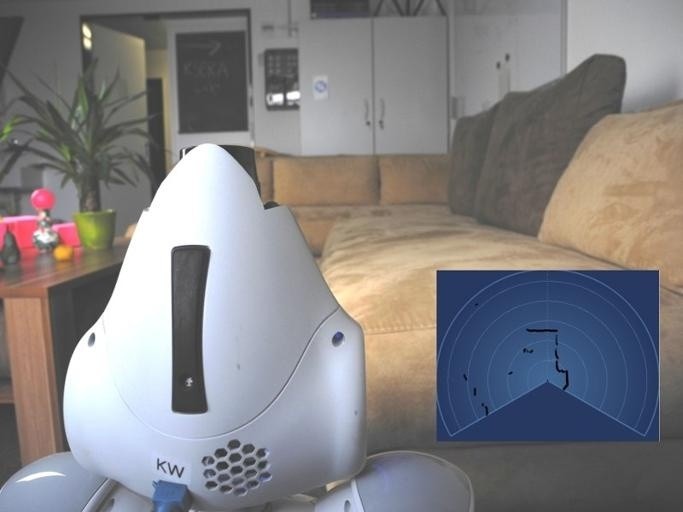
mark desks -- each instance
[0,236,129,467]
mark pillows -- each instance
[475,54,627,235]
[448,101,502,220]
[538,104,682,295]
[378,153,448,204]
[270,155,379,206]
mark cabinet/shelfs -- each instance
[296,14,449,156]
[453,1,564,125]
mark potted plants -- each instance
[0,53,176,250]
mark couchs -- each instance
[253,101,682,512]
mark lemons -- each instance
[53,245,74,262]
